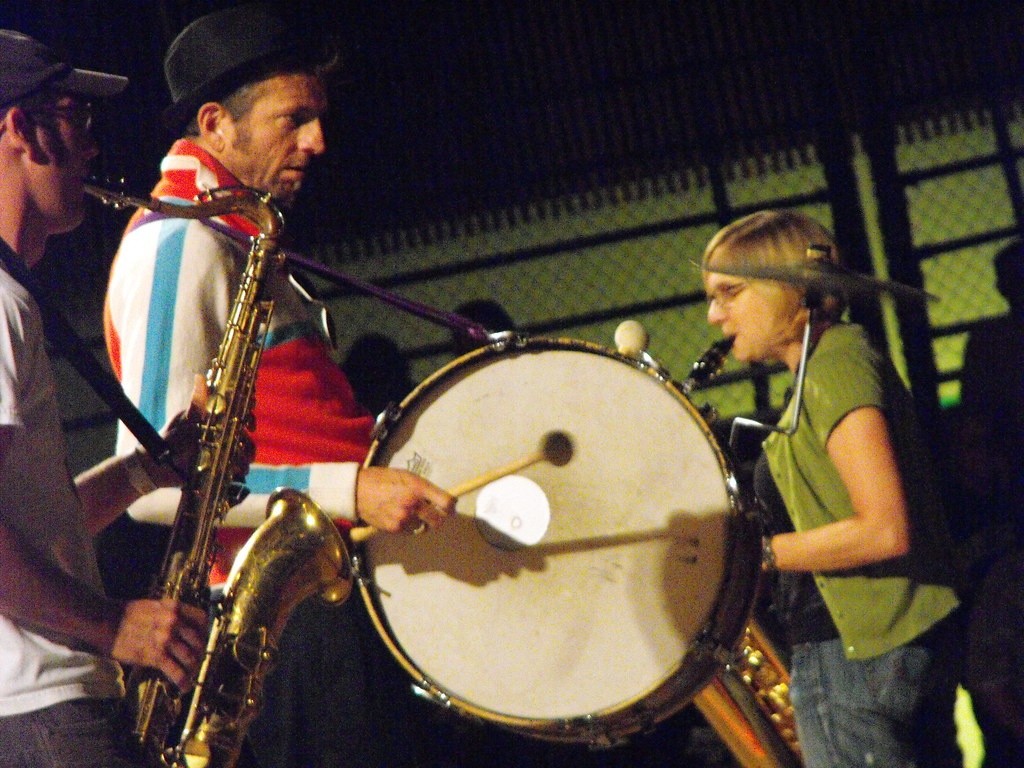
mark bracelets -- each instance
[762,536,781,575]
[120,448,159,495]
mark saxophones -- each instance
[662,336,820,767]
[69,178,352,768]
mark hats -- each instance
[2,31,129,108]
[159,7,340,134]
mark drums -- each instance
[352,334,761,749]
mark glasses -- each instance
[20,100,93,129]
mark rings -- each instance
[410,521,426,537]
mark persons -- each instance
[699,210,1024,768]
[0,12,515,768]
